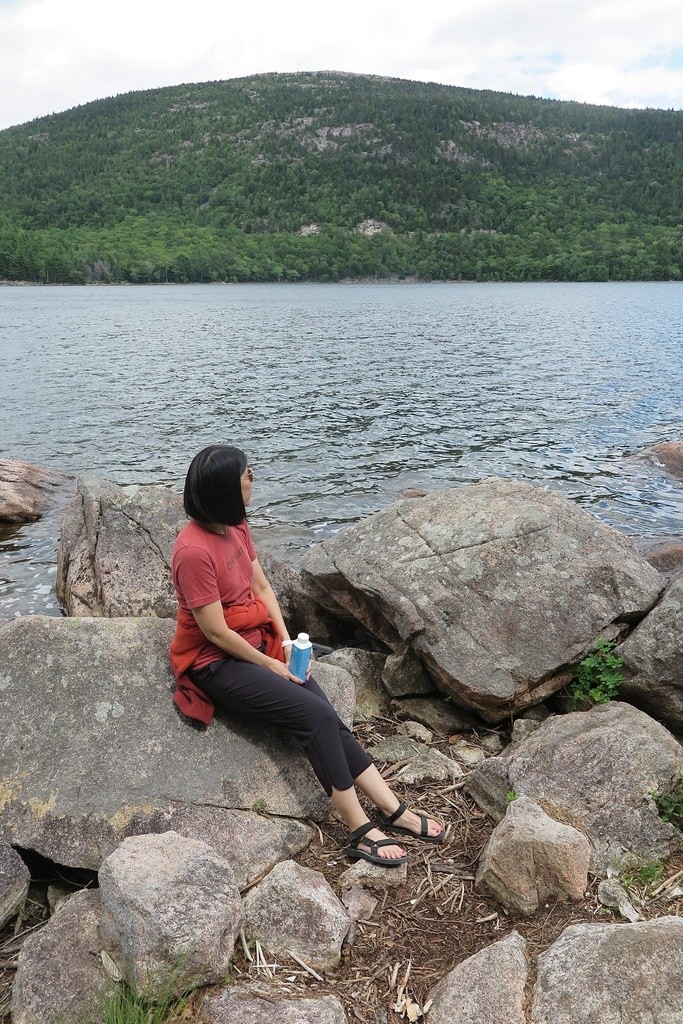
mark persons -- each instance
[169,446,444,865]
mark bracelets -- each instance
[281,640,292,647]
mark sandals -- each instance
[347,822,410,864]
[379,804,445,841]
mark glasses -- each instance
[241,468,254,482]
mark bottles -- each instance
[287,633,313,683]
